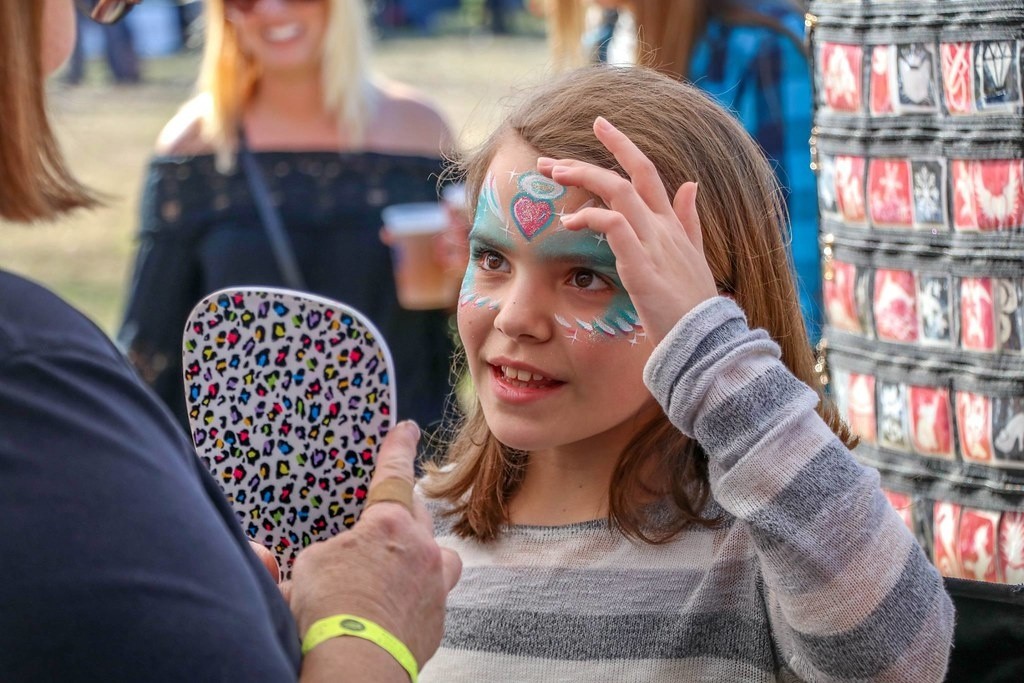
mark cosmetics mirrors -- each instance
[182,285,399,583]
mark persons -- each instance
[527,0,823,365]
[116,0,467,473]
[0,1,461,682]
[412,65,955,679]
